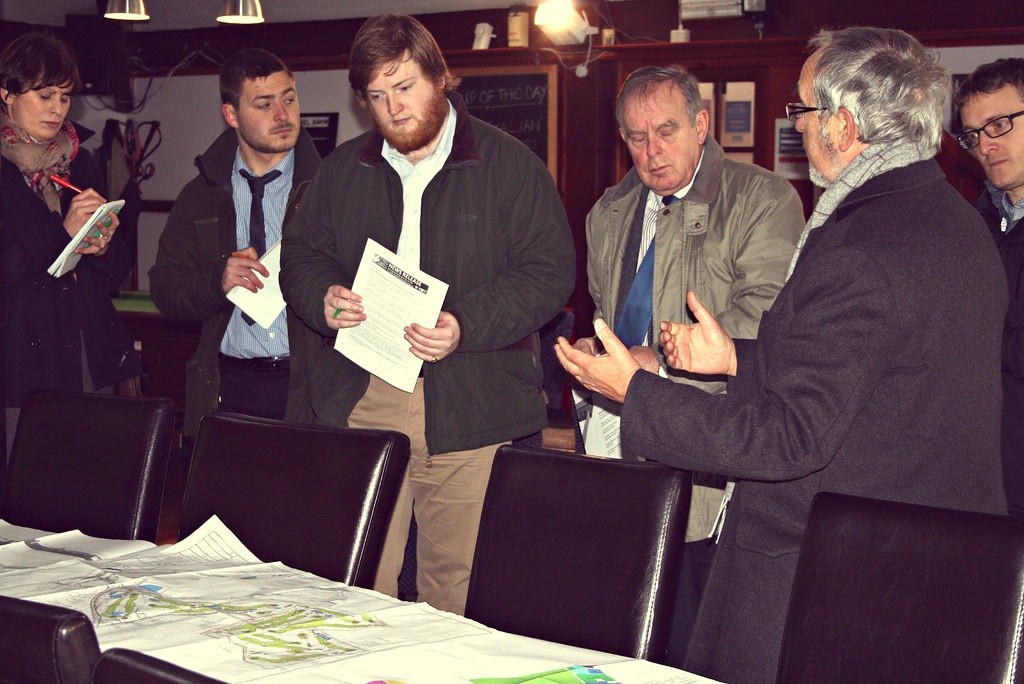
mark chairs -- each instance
[0,395,1024,684]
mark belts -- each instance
[418,366,425,377]
[217,350,294,371]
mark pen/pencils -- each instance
[231,253,249,258]
[333,308,342,319]
[50,175,83,193]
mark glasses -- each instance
[784,102,838,121]
[957,110,1024,150]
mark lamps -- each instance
[102,0,151,20]
[216,0,265,24]
[535,0,599,46]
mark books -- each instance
[47,199,126,278]
[226,238,287,329]
[334,238,449,393]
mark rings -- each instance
[431,355,437,363]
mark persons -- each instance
[572,64,807,601]
[0,32,148,541]
[147,48,322,439]
[952,57,1024,517]
[277,13,579,616]
[553,28,1020,684]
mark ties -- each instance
[234,168,282,326]
[610,193,683,350]
